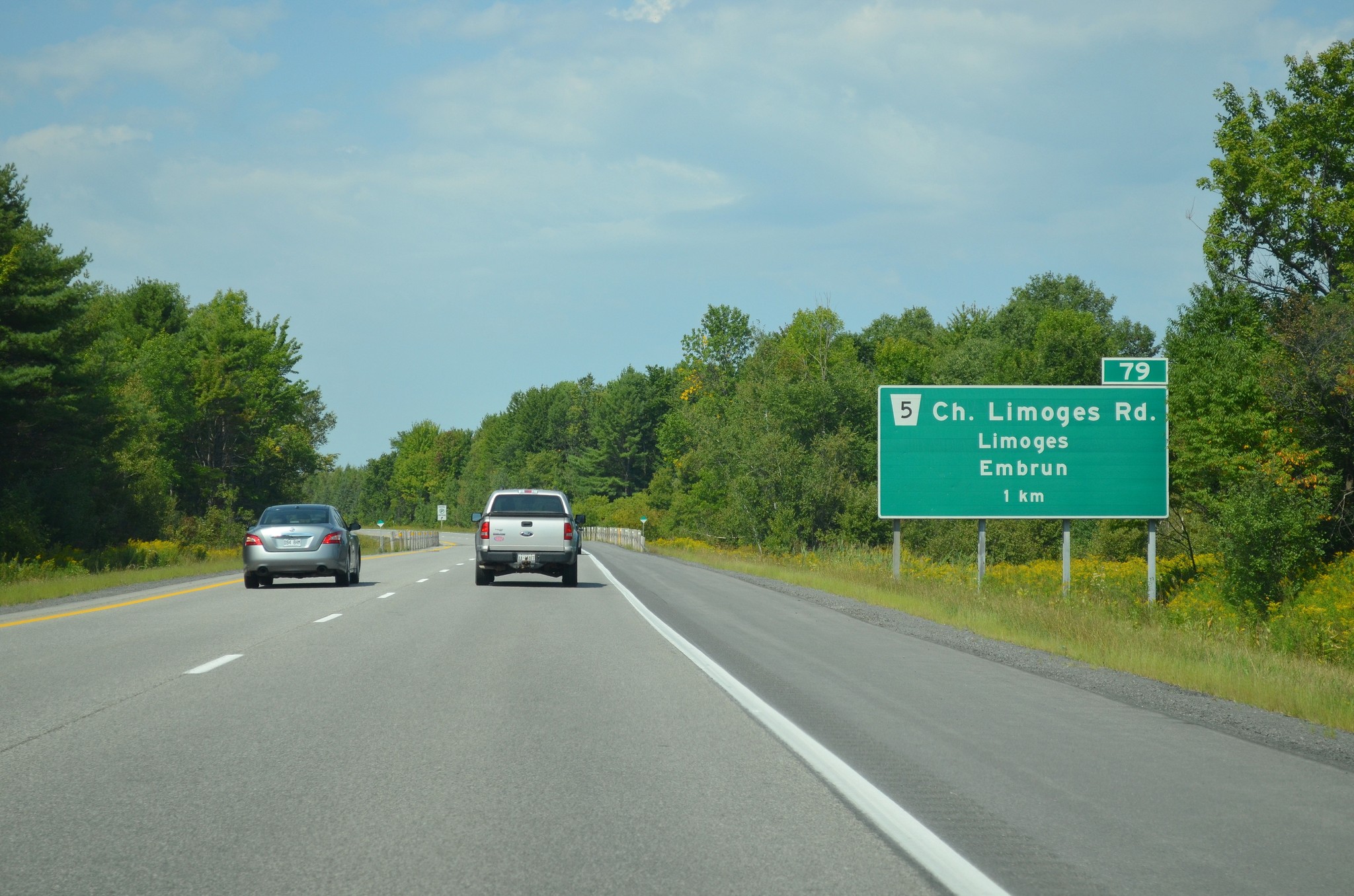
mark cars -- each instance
[575,524,583,555]
[242,504,362,589]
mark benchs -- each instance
[271,515,325,523]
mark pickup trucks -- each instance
[471,489,586,586]
